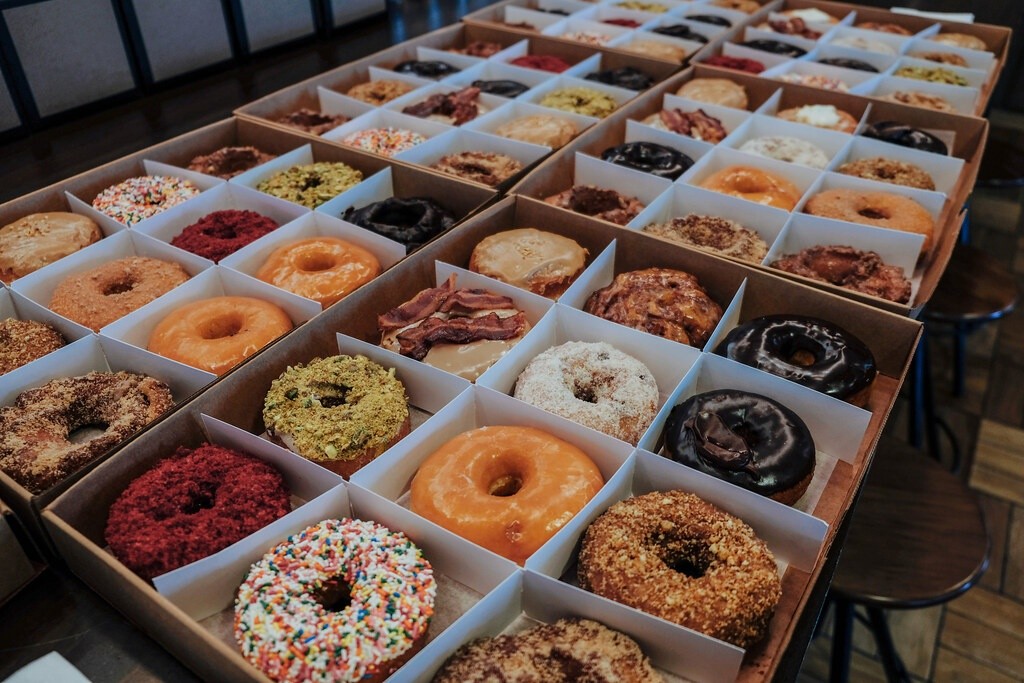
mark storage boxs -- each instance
[0,2,1015,683]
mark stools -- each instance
[904,243,1010,450]
[963,119,1023,241]
[812,436,994,683]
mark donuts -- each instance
[0,0,984,683]
